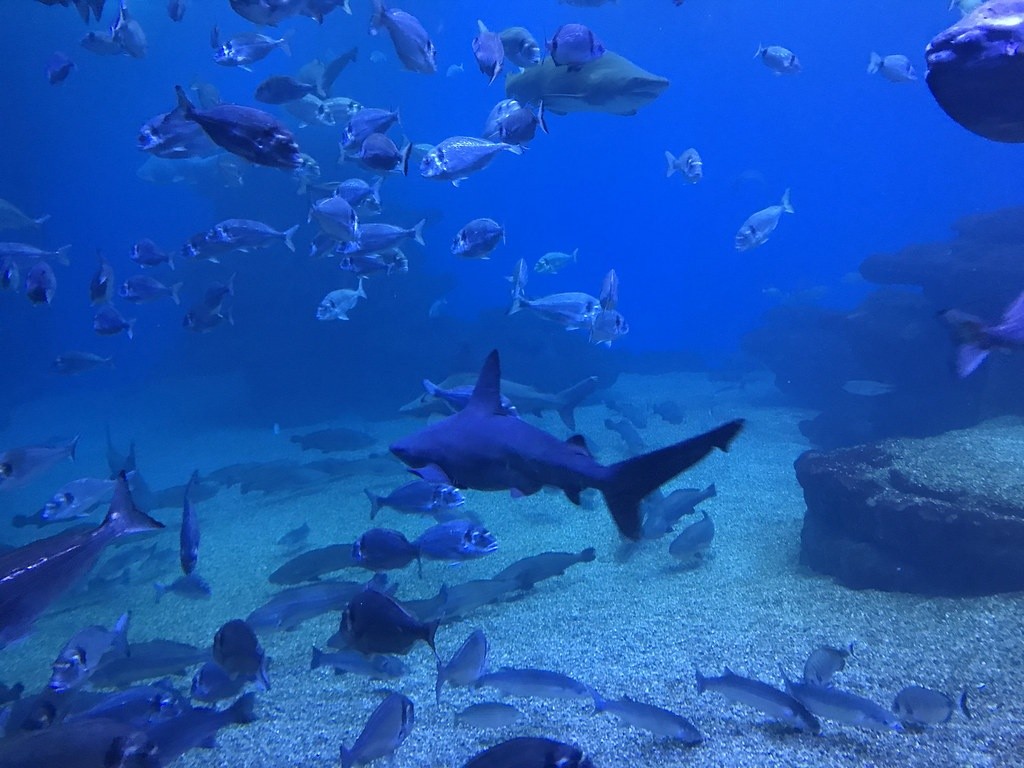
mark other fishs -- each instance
[1,1,1021,767]
